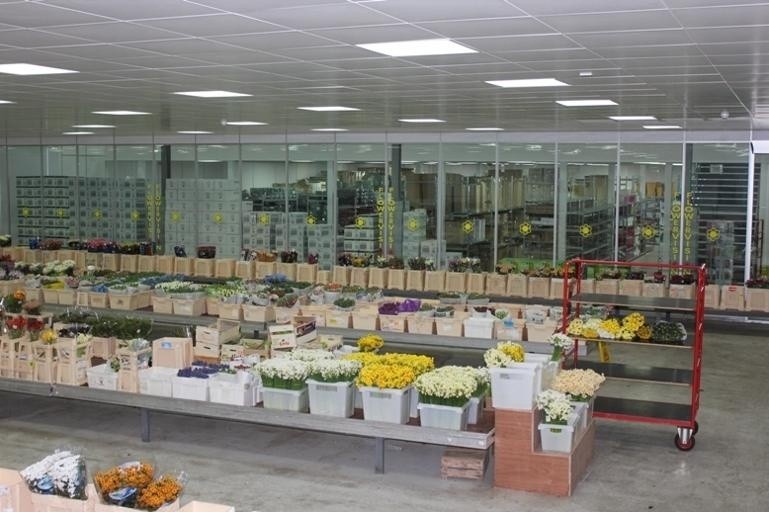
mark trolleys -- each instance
[561,254,707,450]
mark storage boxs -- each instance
[704,284,719,310]
[745,287,769,313]
[537,407,586,453]
[720,285,745,311]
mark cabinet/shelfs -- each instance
[446,174,660,270]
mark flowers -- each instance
[130,475,181,512]
[95,461,153,506]
[22,449,69,494]
[744,278,769,289]
[49,455,88,500]
